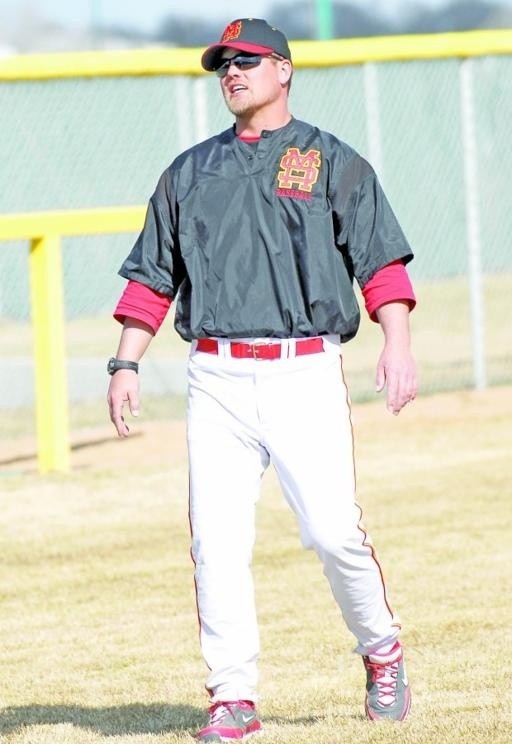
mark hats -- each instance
[200,17,294,73]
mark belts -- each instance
[193,336,329,361]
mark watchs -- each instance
[107,355,138,376]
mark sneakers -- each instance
[195,696,264,744]
[363,650,414,725]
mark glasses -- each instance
[213,52,283,79]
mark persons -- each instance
[105,12,426,743]
